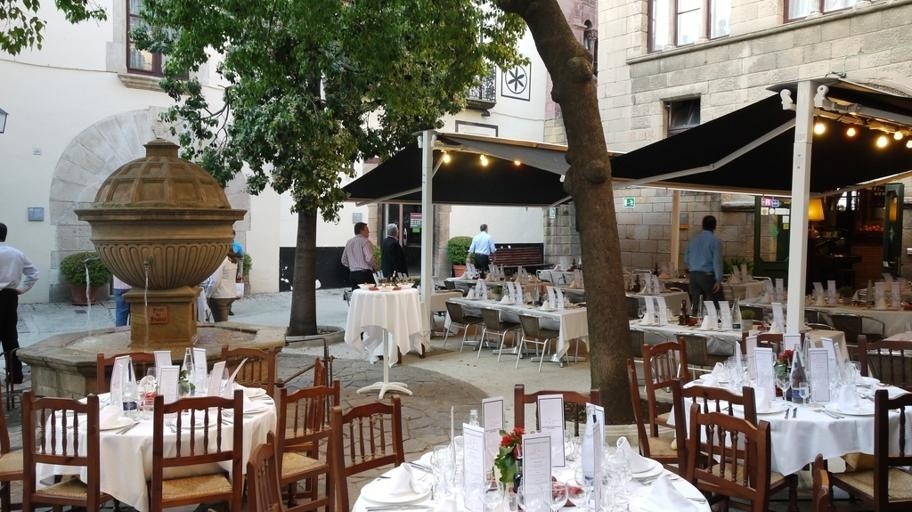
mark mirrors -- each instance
[889,196,897,258]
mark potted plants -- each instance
[447,236,473,277]
[60,252,113,305]
[235,253,253,298]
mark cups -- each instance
[432,446,458,497]
[174,383,191,399]
[776,370,791,406]
[564,479,592,512]
[146,366,162,395]
[574,465,597,512]
[517,482,545,512]
[544,482,569,512]
[119,382,138,419]
[505,492,519,511]
[133,380,148,419]
[463,481,484,512]
[480,480,504,511]
[593,469,620,512]
[798,382,810,410]
[837,384,860,410]
[609,458,633,505]
[845,361,861,387]
[447,435,462,472]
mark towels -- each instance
[641,476,698,511]
[540,301,549,310]
[100,403,121,427]
[501,295,509,304]
[242,394,258,411]
[189,409,211,424]
[245,386,267,397]
[770,322,781,333]
[466,288,474,298]
[702,316,714,330]
[388,463,421,494]
[641,313,648,324]
[615,437,657,474]
[839,384,861,412]
[711,362,729,381]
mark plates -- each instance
[80,416,133,431]
[825,400,875,416]
[361,469,433,503]
[730,405,788,414]
[418,450,434,469]
[171,414,219,429]
[632,456,665,479]
[699,373,729,383]
[244,388,268,399]
[225,404,269,416]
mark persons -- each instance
[466,224,497,280]
[684,215,725,316]
[381,223,409,277]
[0,223,40,386]
[113,272,132,328]
[341,223,376,291]
[232,229,243,258]
[204,244,242,323]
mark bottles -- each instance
[122,383,136,420]
[500,263,505,281]
[791,344,806,403]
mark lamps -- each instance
[893,127,902,141]
[877,131,887,147]
[906,137,912,149]
[813,116,825,135]
[807,199,825,238]
[846,123,855,138]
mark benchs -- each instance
[493,243,554,276]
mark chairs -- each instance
[0,401,62,512]
[284,357,326,512]
[685,403,799,511]
[671,378,800,512]
[536,270,541,279]
[805,310,834,329]
[739,305,768,323]
[221,345,275,398]
[858,335,912,391]
[514,384,602,437]
[274,380,340,511]
[477,307,528,362]
[641,336,689,437]
[828,312,886,360]
[97,352,154,394]
[515,315,570,373]
[331,395,405,512]
[675,334,731,380]
[631,329,672,393]
[247,430,286,512]
[828,390,912,512]
[628,357,712,502]
[146,390,243,512]
[443,281,464,294]
[22,390,120,512]
[684,403,772,511]
[444,302,490,353]
[742,332,805,355]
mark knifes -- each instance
[784,408,789,420]
[364,504,432,511]
[792,407,797,419]
